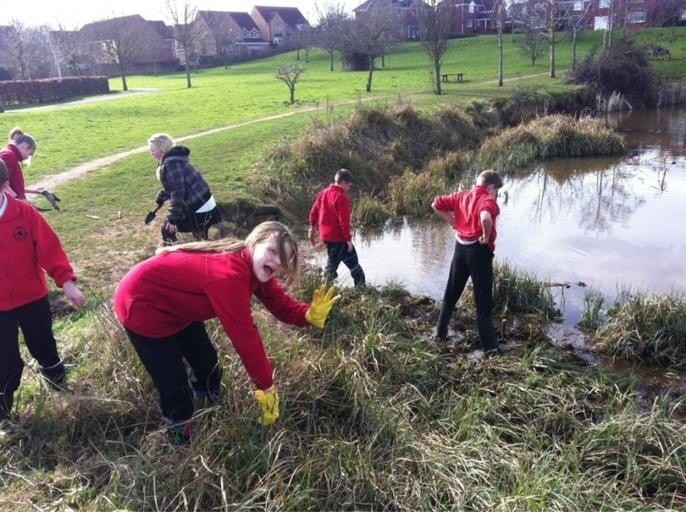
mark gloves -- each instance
[305,284,342,329]
[254,385,280,426]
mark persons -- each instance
[0,158,85,421]
[149,133,222,247]
[307,168,368,292]
[428,169,508,357]
[109,220,344,448]
[0,128,47,203]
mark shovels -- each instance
[145,207,159,224]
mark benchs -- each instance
[440,72,465,82]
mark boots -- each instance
[0,356,74,423]
